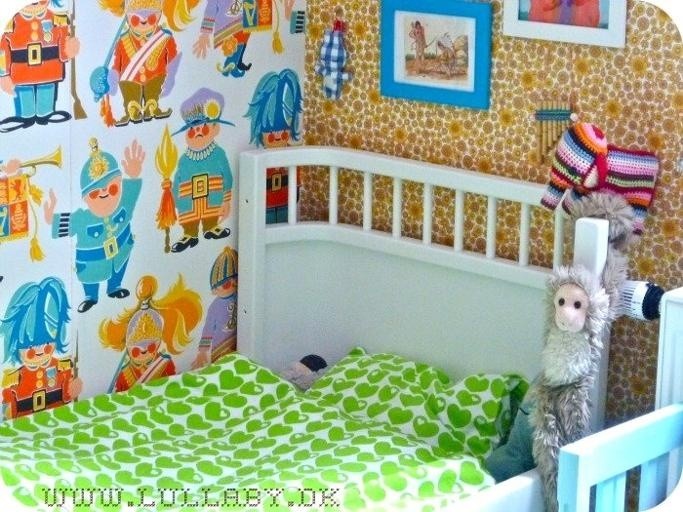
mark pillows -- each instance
[304,344,525,459]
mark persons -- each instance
[409,20,427,74]
[437,40,456,80]
[523,193,635,512]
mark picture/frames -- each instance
[377,0,495,112]
[502,2,631,54]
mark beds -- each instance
[1,144,620,512]
